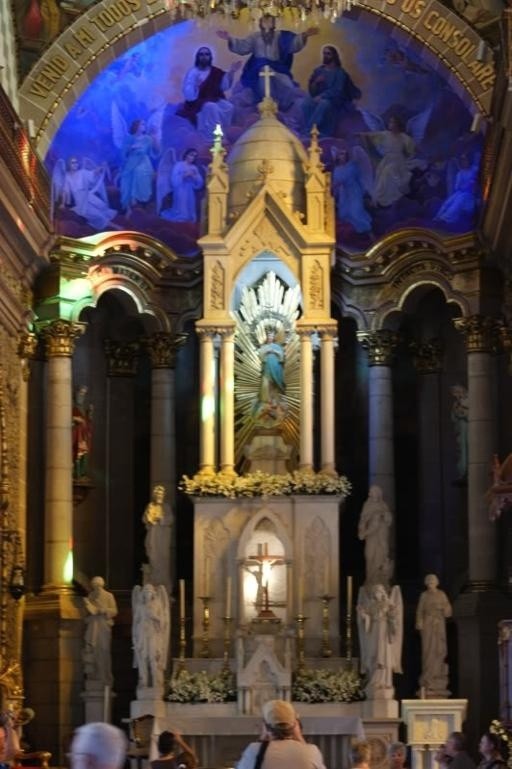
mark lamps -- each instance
[9,566,27,602]
[164,1,356,30]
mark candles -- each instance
[346,575,353,617]
[179,578,186,620]
[324,558,331,595]
[225,576,232,617]
[204,556,212,596]
[296,576,305,617]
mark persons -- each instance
[257,325,285,403]
[80,576,119,686]
[151,729,198,769]
[356,583,406,688]
[142,485,175,581]
[130,583,170,686]
[348,737,371,769]
[68,721,128,769]
[444,731,476,769]
[235,698,327,768]
[0,720,11,768]
[387,741,406,769]
[476,732,507,769]
[357,484,393,572]
[414,573,452,690]
[435,743,453,769]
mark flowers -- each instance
[165,670,235,703]
[179,469,353,499]
[293,667,364,703]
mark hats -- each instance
[257,698,297,735]
